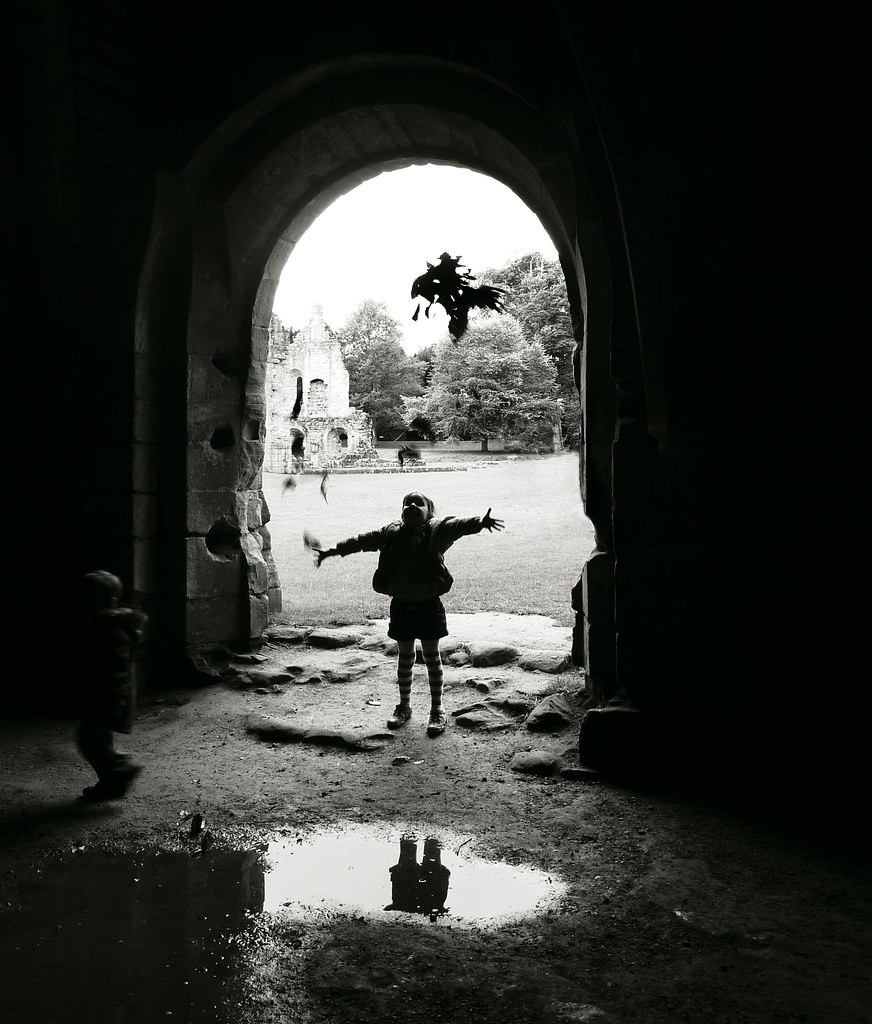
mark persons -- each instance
[75,570,144,799]
[312,491,505,734]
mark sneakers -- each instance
[388,705,411,727]
[427,710,446,734]
